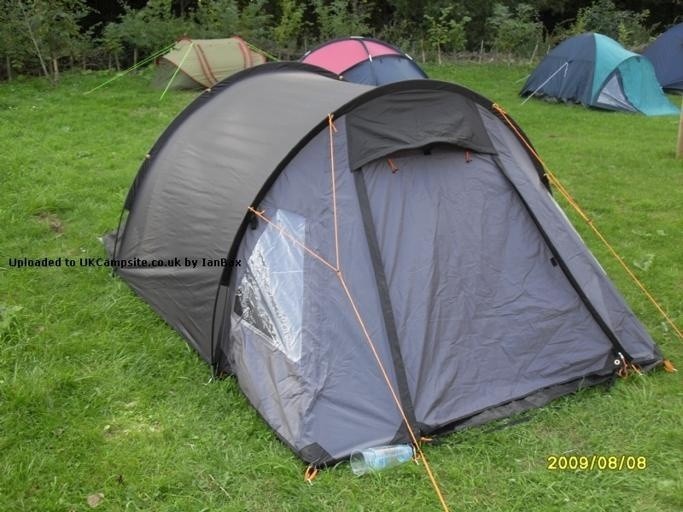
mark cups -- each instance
[349,443,412,477]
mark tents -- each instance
[517,31,681,116]
[297,36,430,87]
[110,58,666,480]
[148,37,266,94]
[639,23,682,93]
